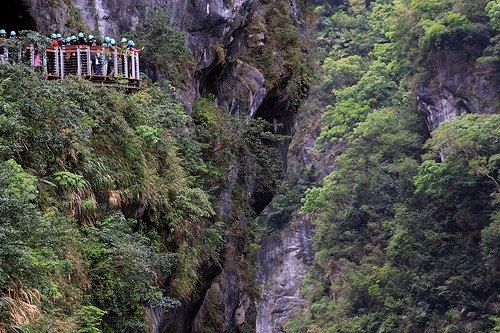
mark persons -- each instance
[0,28,146,79]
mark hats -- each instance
[50,33,136,48]
[10,31,16,36]
[0,29,6,34]
